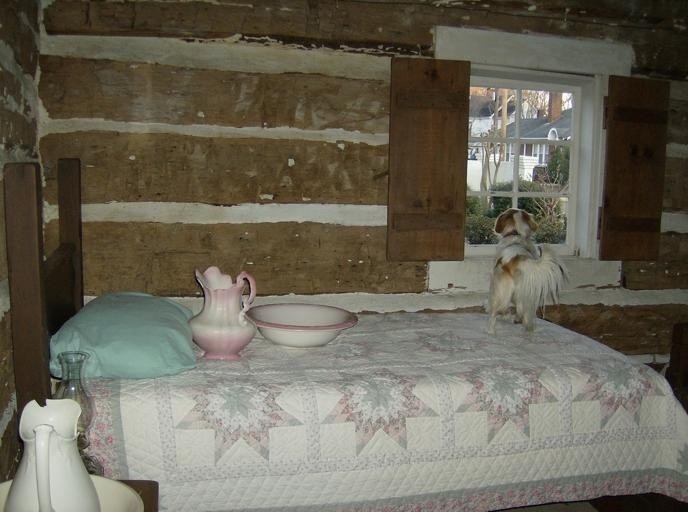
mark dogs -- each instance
[485,207,570,338]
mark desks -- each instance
[117,477,161,512]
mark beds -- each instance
[0,157,687,511]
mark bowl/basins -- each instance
[2,472,145,512]
[248,302,360,348]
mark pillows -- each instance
[47,290,203,384]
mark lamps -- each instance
[52,351,100,475]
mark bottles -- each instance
[48,353,98,445]
